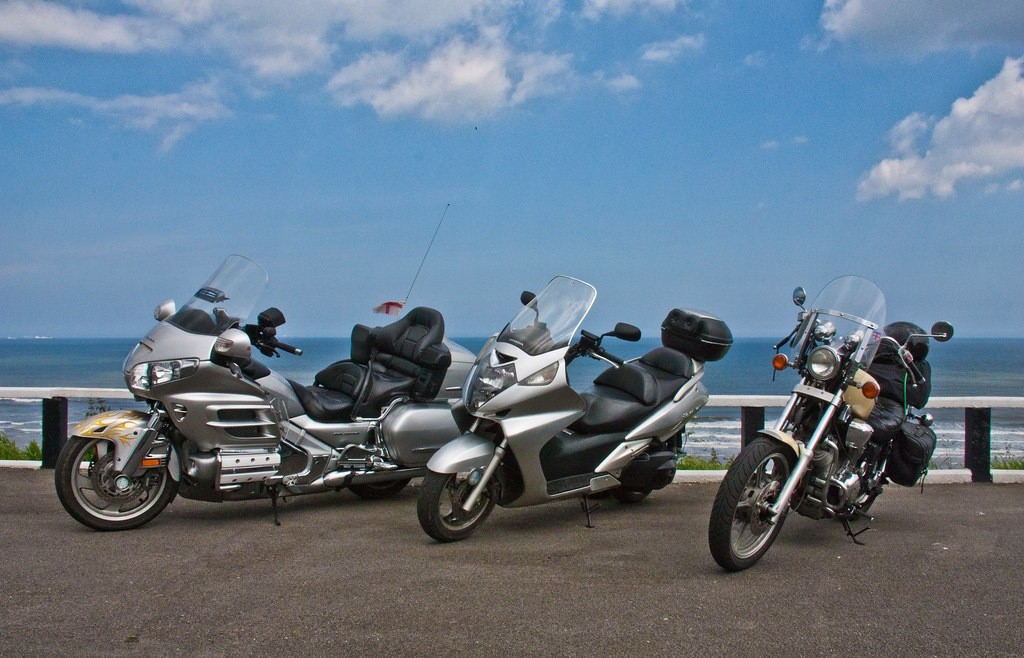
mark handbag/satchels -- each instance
[887,417,937,494]
[868,346,931,410]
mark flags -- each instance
[872,331,882,342]
[372,298,406,319]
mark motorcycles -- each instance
[707,272,955,573]
[53,252,483,531]
[417,273,735,542]
